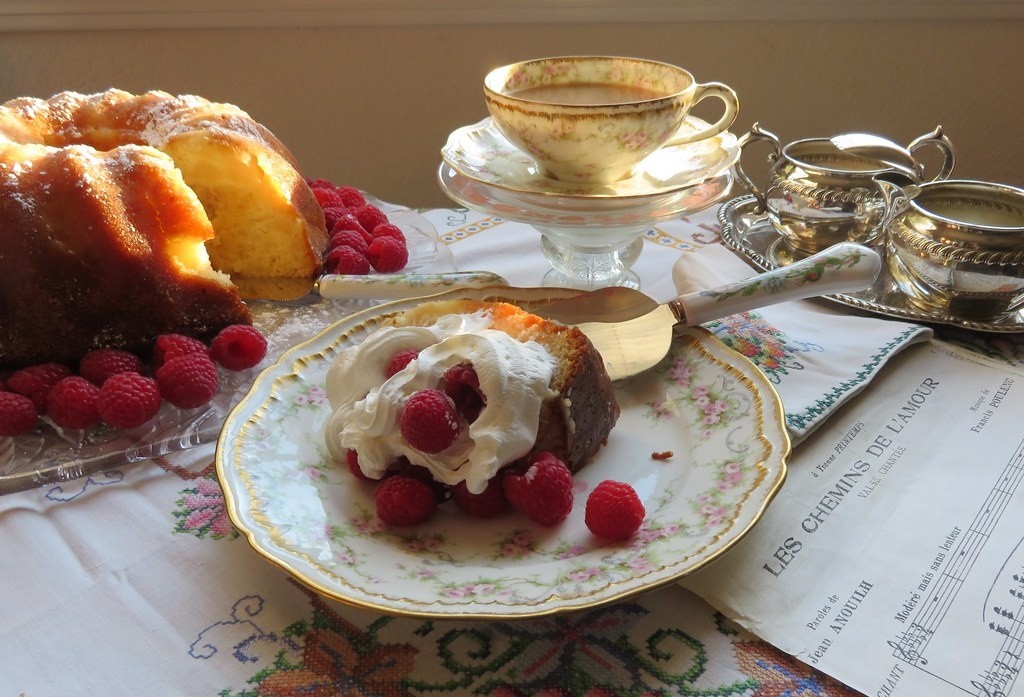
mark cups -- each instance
[870,169,1024,324]
[727,120,959,256]
[482,52,741,189]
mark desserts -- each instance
[319,299,617,493]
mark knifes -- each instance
[228,269,522,302]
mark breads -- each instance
[0,88,328,366]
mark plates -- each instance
[0,181,460,489]
[439,112,742,200]
[214,283,792,621]
[435,158,734,230]
